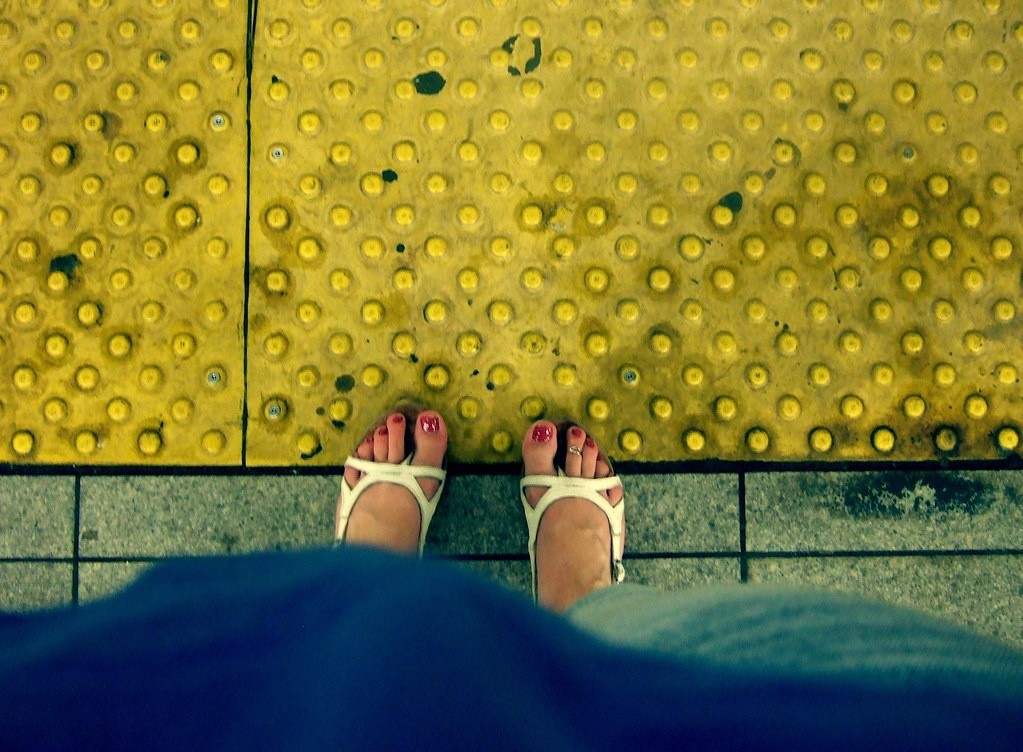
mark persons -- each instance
[0,409,1023,752]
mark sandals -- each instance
[333,404,447,561]
[519,420,626,612]
